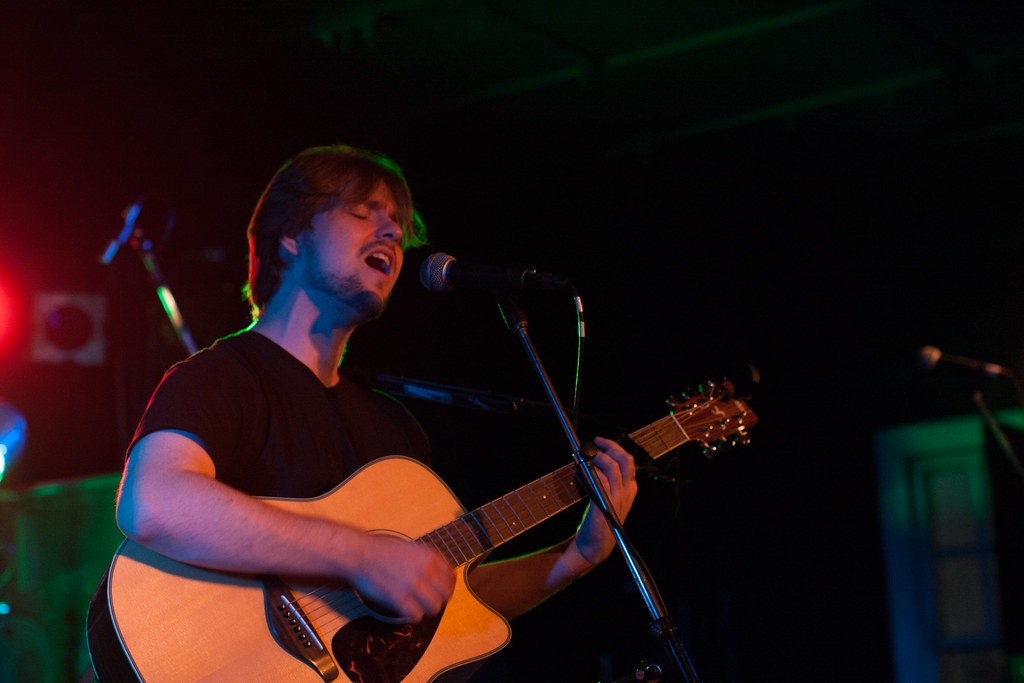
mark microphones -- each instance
[920,347,1009,376]
[100,203,142,263]
[418,252,568,296]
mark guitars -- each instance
[82,365,760,683]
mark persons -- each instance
[114,146,638,622]
